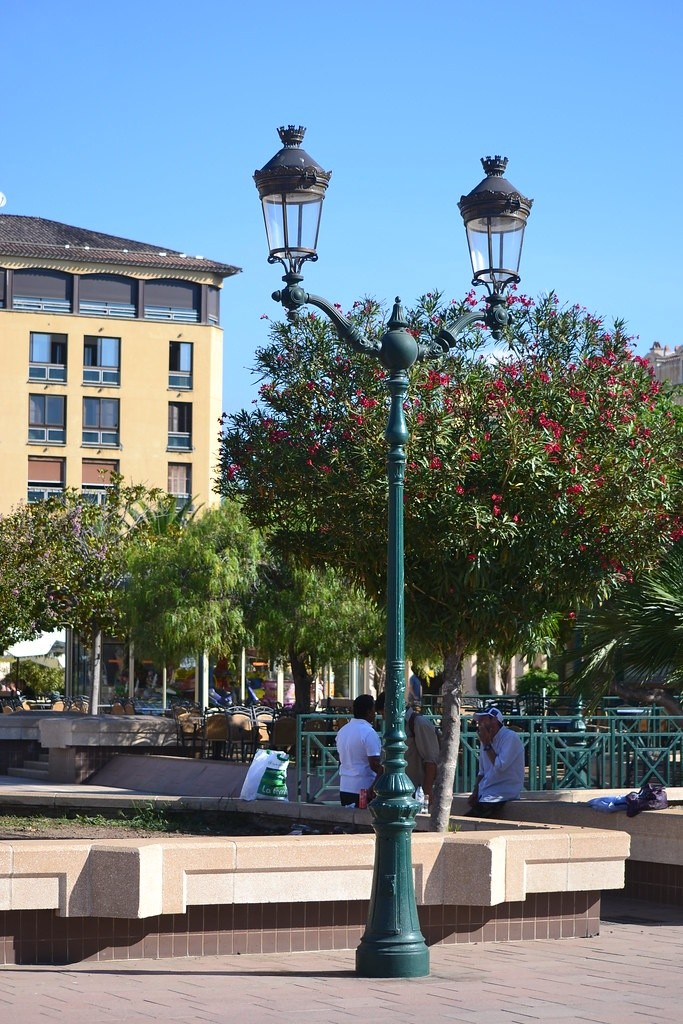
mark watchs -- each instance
[483,744,492,751]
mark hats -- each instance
[473,707,503,726]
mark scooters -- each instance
[208,678,261,708]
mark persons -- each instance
[407,675,422,706]
[334,694,384,806]
[375,691,441,798]
[462,707,526,819]
[16,679,35,700]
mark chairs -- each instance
[0,689,562,775]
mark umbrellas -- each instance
[0,624,66,693]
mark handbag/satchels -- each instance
[240,748,290,800]
[625,782,668,817]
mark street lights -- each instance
[252,124,534,975]
